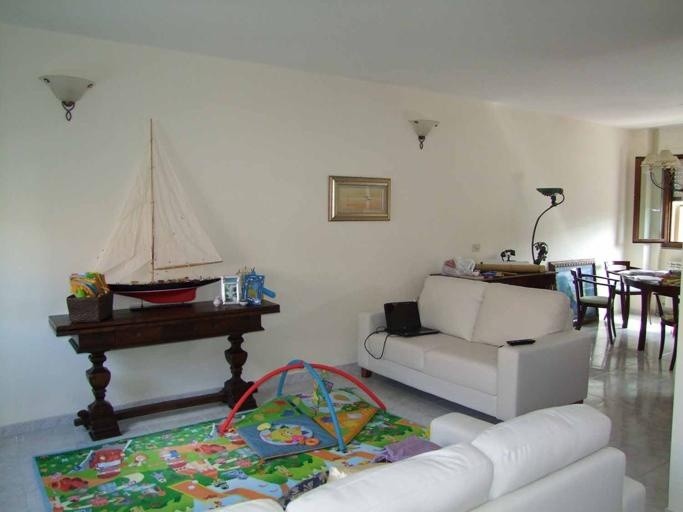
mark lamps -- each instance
[532,188,565,265]
[639,151,683,193]
[411,119,440,150]
[39,74,96,121]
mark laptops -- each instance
[383,302,439,337]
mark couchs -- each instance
[358,275,597,422]
[198,413,647,512]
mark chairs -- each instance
[577,267,620,324]
[653,292,679,371]
[603,261,652,325]
[572,271,617,344]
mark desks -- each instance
[431,272,556,290]
[47,301,281,440]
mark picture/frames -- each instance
[223,282,239,305]
[548,258,599,326]
[328,175,392,220]
[221,275,238,301]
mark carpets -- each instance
[31,407,429,512]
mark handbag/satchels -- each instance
[441,257,479,277]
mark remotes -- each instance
[507,339,535,345]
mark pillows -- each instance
[475,282,570,346]
[470,405,611,501]
[418,274,487,342]
[287,442,493,512]
[202,498,284,511]
